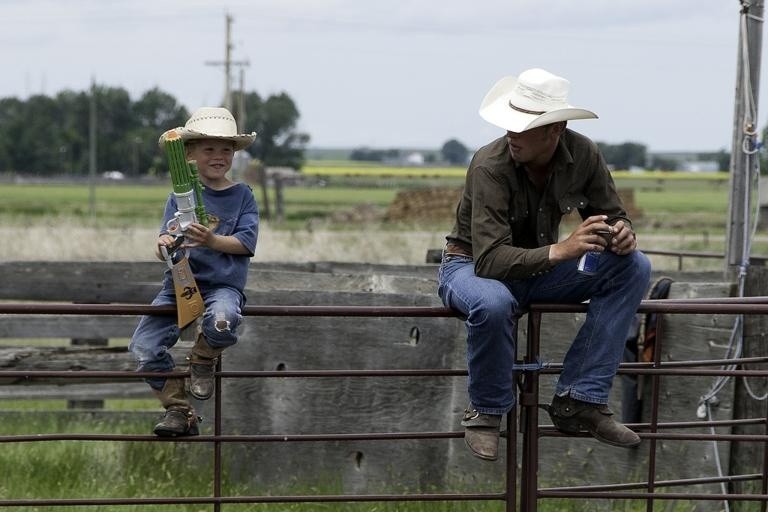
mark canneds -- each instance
[575,230,601,276]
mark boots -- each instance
[144,367,200,437]
[189,331,227,402]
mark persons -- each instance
[437,68,652,462]
[128,106,260,437]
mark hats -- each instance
[158,106,257,151]
[476,68,598,134]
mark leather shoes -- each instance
[552,391,642,446]
[461,400,502,461]
